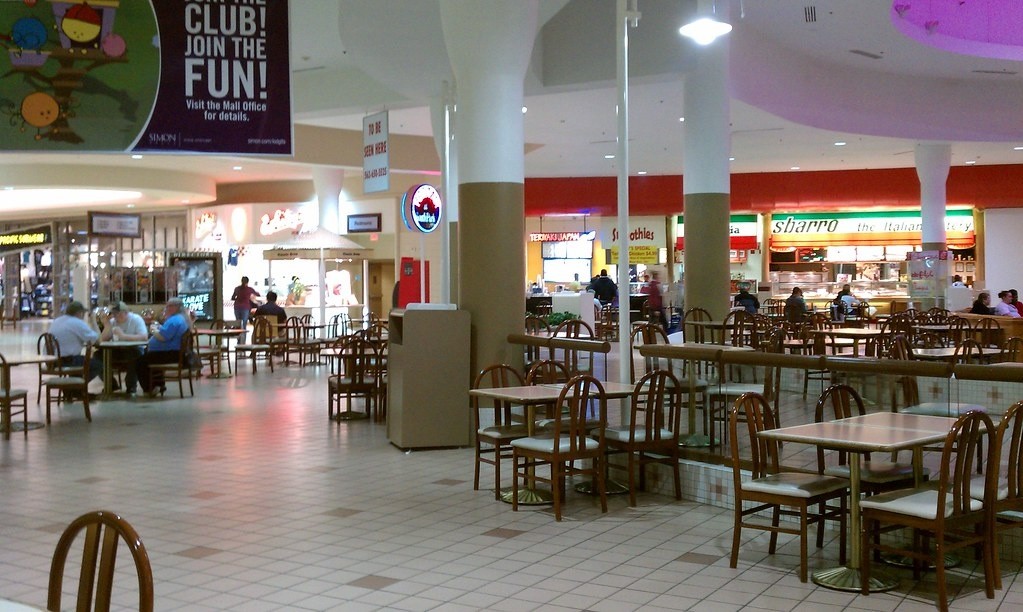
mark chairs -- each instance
[0,296,1023,611]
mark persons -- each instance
[94,301,148,392]
[231,276,260,344]
[586,269,617,306]
[834,284,860,314]
[784,287,813,325]
[641,271,668,331]
[731,284,760,321]
[972,289,1023,318]
[953,275,967,287]
[43,302,102,399]
[250,292,287,355]
[136,298,191,396]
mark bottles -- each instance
[140,306,167,332]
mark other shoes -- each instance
[144,386,161,398]
[101,384,119,394]
[126,390,135,399]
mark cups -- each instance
[952,254,973,260]
[112,326,120,341]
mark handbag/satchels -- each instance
[183,348,201,369]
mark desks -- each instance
[349,318,386,333]
[320,347,388,379]
[909,325,1002,348]
[192,327,248,379]
[753,411,956,592]
[830,305,860,322]
[904,343,1020,363]
[95,340,151,403]
[543,380,664,497]
[0,352,58,432]
[597,309,638,337]
[827,412,1009,572]
[684,320,756,348]
[809,329,894,360]
[757,304,785,316]
[468,381,595,508]
[267,323,325,367]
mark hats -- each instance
[112,300,128,311]
